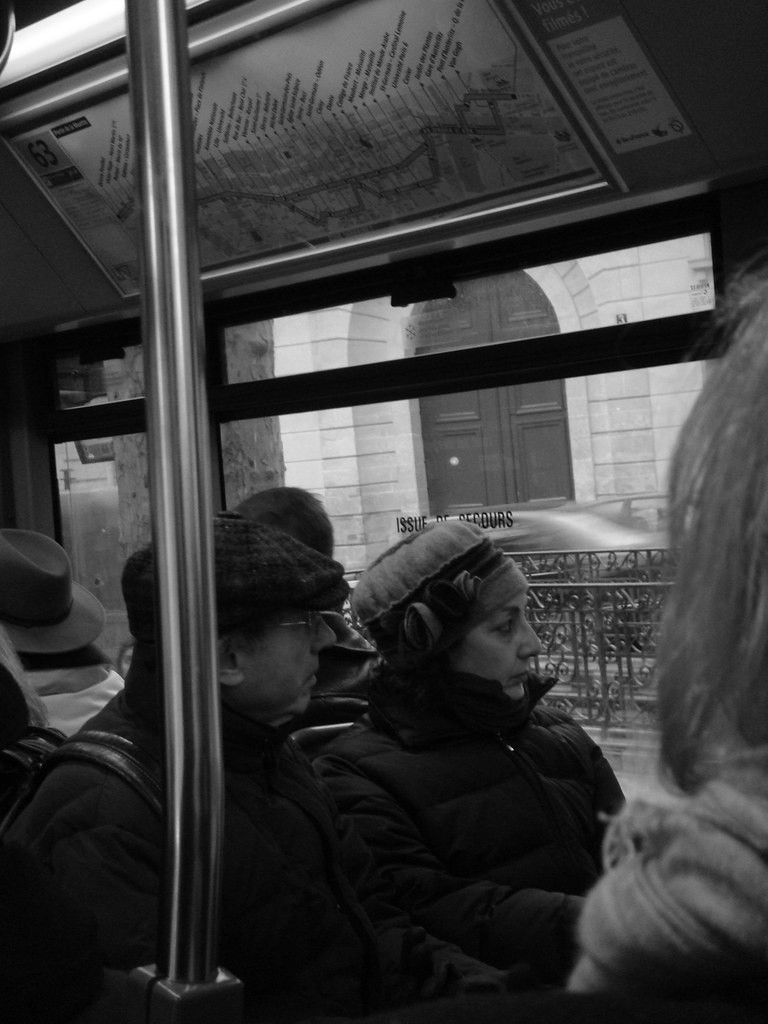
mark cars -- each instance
[359,503,672,613]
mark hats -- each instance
[122,511,352,641]
[0,528,107,654]
[350,519,530,670]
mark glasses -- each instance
[261,610,320,635]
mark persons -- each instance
[313,522,628,983]
[0,487,503,1024]
[564,256,768,990]
